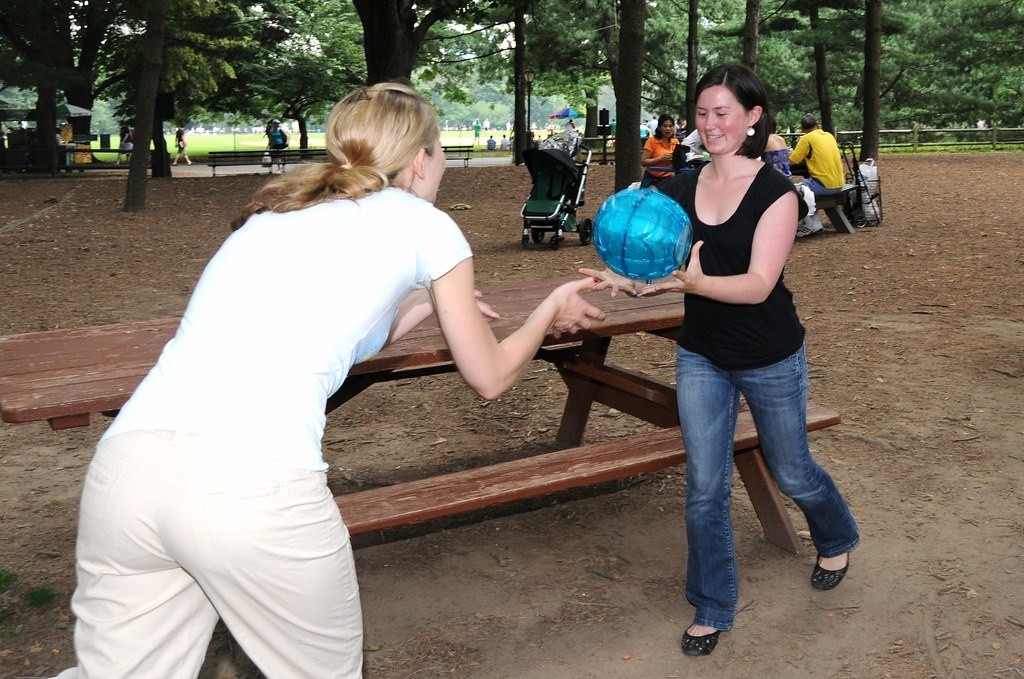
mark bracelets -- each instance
[625,278,651,299]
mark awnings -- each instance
[0,97,36,121]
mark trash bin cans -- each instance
[59,140,76,166]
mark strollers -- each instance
[520,142,592,245]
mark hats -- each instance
[272,119,281,124]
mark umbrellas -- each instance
[550,103,588,119]
[56,102,92,118]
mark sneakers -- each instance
[796,215,823,238]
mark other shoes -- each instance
[680,622,721,656]
[811,551,849,590]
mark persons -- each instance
[268,119,290,176]
[577,62,861,659]
[172,126,193,166]
[114,118,136,166]
[472,113,844,241]
[56,126,63,145]
[53,80,608,679]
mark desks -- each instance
[649,167,696,173]
[0,270,800,554]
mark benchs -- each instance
[335,402,841,535]
[207,149,329,177]
[443,145,475,168]
[815,183,859,235]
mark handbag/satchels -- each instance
[128,131,135,140]
[859,157,878,190]
[178,141,187,148]
[261,146,272,164]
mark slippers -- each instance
[172,163,178,166]
[189,162,195,165]
